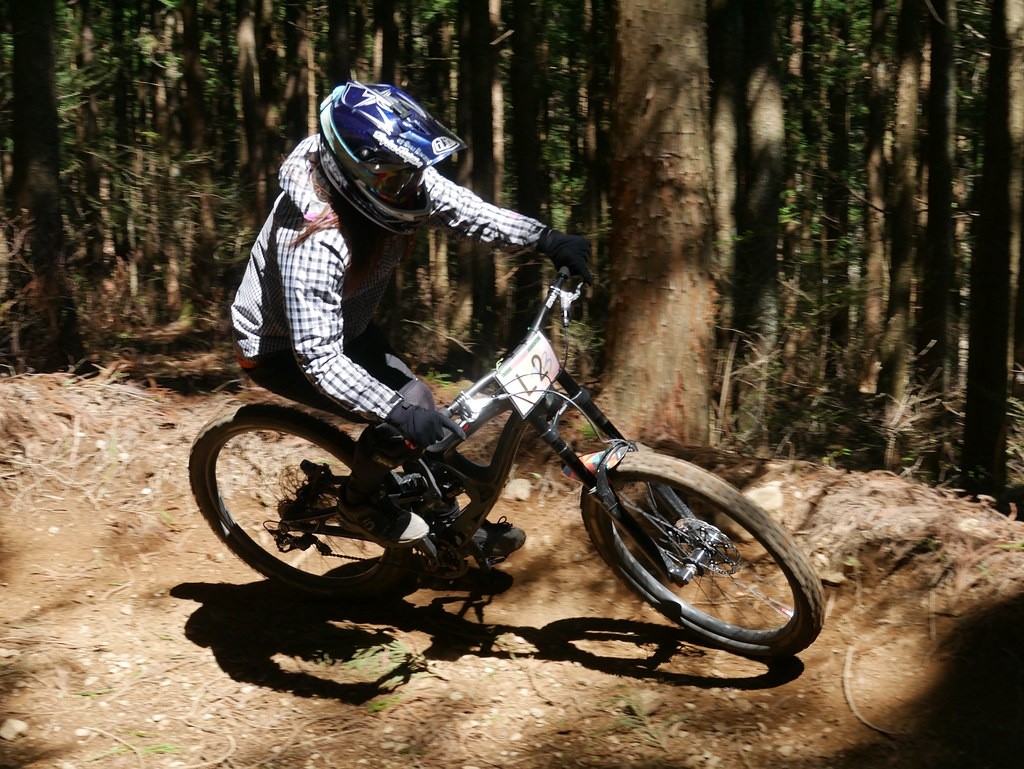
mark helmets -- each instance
[318,79,467,235]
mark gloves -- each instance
[384,400,466,450]
[537,230,594,287]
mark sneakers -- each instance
[458,516,526,558]
[337,495,430,548]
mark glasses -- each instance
[321,94,425,202]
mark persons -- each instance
[231,81,594,554]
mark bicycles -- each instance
[188,266,829,663]
